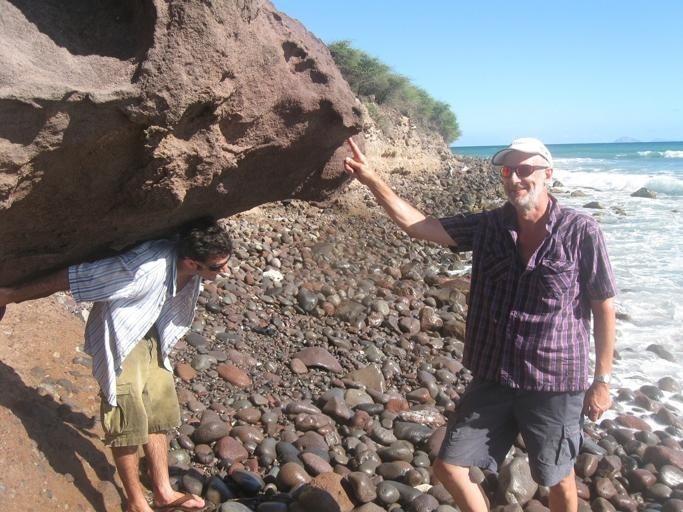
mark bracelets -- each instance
[593,373,610,384]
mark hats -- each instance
[491,137,553,166]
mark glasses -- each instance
[499,165,548,178]
[202,258,230,272]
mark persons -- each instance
[0,217,235,512]
[342,126,621,512]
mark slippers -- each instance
[152,492,208,512]
